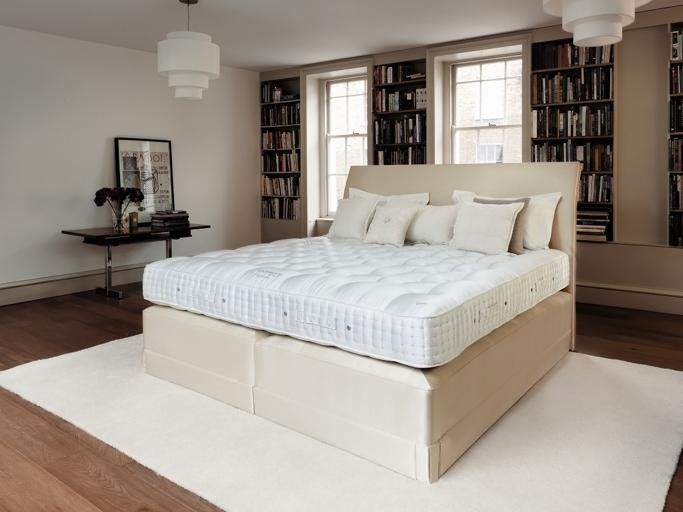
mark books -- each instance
[370,65,427,165]
[529,40,615,245]
[261,82,300,220]
[665,23,682,245]
[149,209,190,228]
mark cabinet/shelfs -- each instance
[530,38,614,244]
[258,76,301,220]
[370,58,428,165]
[668,21,683,248]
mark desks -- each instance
[60,223,209,300]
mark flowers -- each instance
[93,187,143,233]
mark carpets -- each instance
[0,330,683,512]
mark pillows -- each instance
[325,198,377,241]
[363,204,416,247]
[449,188,562,250]
[406,206,453,244]
[344,185,431,207]
[471,194,531,256]
[446,199,526,255]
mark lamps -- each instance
[542,0,653,49]
[156,0,220,101]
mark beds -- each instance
[141,160,584,486]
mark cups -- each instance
[271,90,280,103]
[129,212,137,227]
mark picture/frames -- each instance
[115,136,174,227]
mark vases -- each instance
[111,209,127,232]
